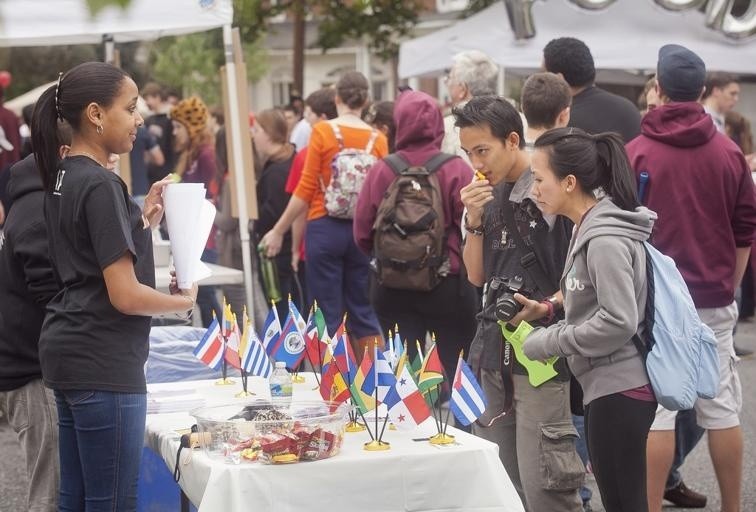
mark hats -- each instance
[167,95,208,141]
[658,44,706,95]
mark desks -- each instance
[155,263,244,332]
[143,373,527,511]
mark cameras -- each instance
[484,274,535,322]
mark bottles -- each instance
[269,362,292,399]
[256,243,282,305]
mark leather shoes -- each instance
[663,482,707,508]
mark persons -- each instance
[1,35,755,512]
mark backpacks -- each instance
[317,120,378,220]
[584,240,722,411]
[368,151,462,294]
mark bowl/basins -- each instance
[191,401,353,463]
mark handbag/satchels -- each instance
[571,376,587,415]
